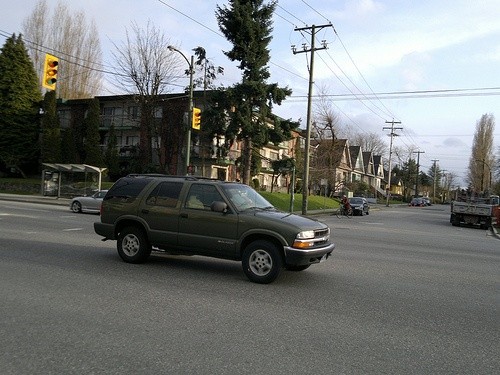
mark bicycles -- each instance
[336,203,352,219]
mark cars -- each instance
[69,189,108,213]
[408,197,432,207]
[341,197,370,216]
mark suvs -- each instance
[94,174,336,285]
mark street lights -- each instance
[167,45,194,175]
[475,159,492,188]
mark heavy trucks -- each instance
[450,189,493,230]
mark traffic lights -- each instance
[42,54,59,91]
[192,107,201,131]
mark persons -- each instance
[229,188,255,208]
[340,195,350,217]
